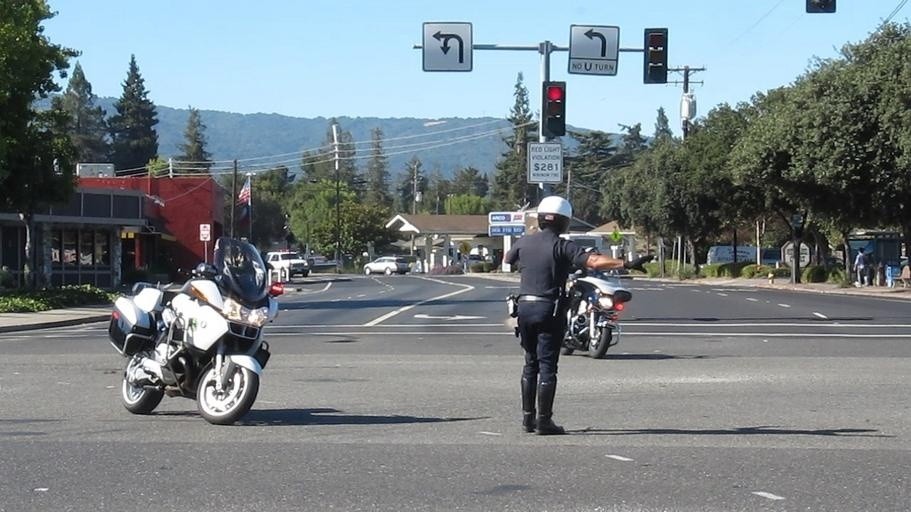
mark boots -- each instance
[537,381,565,435]
[522,376,537,431]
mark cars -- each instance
[266,252,309,278]
[363,257,410,276]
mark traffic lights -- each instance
[807,1,839,12]
[541,81,566,137]
[643,28,667,83]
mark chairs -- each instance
[892,265,911,289]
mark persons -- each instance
[853,246,868,288]
[504,194,656,438]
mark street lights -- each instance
[310,179,369,274]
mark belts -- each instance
[518,295,556,303]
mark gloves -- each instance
[623,256,654,273]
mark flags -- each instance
[234,174,250,207]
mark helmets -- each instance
[537,195,572,233]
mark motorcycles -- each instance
[560,270,632,358]
[108,237,283,424]
